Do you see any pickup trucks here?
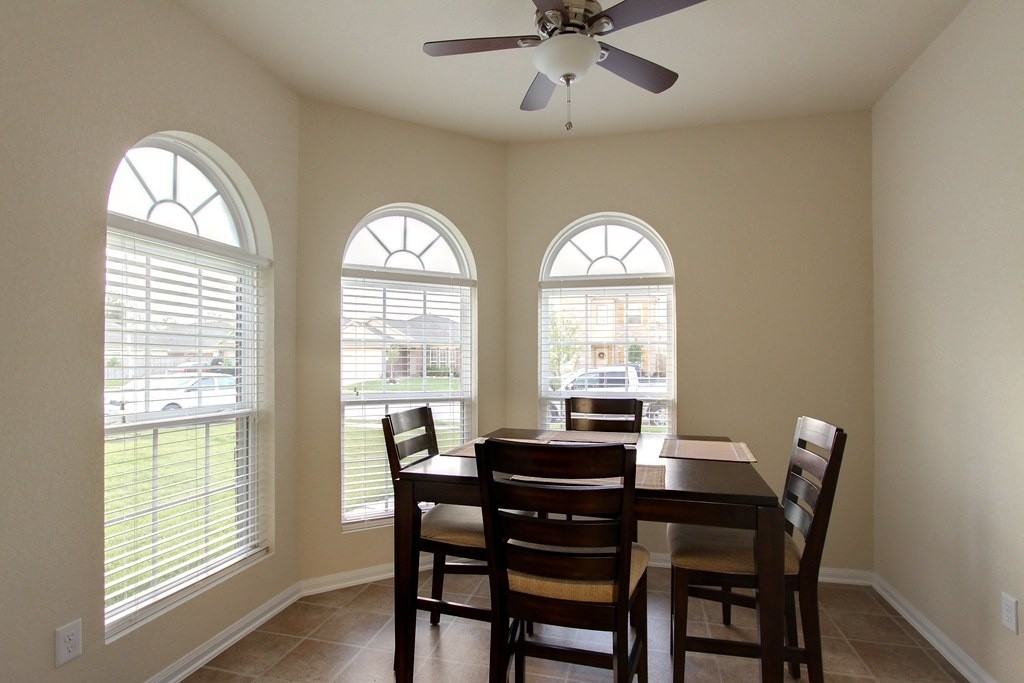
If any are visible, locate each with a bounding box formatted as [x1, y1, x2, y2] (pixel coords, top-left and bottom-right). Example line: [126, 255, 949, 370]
[548, 366, 673, 427]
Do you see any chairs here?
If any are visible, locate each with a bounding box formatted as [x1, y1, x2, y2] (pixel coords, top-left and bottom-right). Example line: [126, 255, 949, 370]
[473, 437, 650, 683]
[382, 407, 533, 670]
[666, 416, 847, 683]
[538, 395, 643, 542]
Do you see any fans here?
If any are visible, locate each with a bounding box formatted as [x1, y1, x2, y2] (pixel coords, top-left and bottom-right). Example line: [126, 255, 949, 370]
[421, 0, 708, 111]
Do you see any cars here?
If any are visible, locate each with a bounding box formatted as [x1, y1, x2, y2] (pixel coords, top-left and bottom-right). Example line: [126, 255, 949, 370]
[124, 358, 235, 414]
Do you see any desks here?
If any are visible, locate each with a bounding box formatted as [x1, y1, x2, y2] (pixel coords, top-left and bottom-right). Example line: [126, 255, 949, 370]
[396, 427, 786, 683]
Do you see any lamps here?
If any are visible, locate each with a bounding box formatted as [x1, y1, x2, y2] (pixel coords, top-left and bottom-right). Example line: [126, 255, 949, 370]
[533, 33, 601, 132]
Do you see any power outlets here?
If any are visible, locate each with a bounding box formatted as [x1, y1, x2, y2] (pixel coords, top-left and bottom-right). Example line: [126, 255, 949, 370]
[1001, 592, 1018, 633]
